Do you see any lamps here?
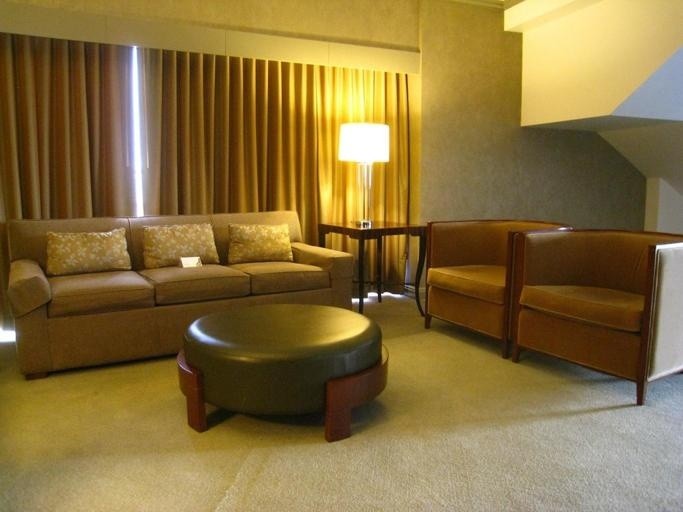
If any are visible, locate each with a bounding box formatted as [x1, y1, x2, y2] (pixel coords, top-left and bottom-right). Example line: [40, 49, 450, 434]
[339, 124, 391, 224]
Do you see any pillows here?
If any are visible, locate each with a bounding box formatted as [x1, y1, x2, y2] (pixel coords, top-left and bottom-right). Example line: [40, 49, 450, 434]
[45, 225, 131, 276]
[227, 222, 295, 263]
[142, 221, 220, 269]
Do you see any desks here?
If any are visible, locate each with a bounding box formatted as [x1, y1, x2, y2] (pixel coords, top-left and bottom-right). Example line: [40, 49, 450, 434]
[175, 302, 391, 444]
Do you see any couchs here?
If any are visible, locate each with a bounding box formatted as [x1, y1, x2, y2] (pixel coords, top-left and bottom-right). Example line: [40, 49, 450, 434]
[426, 218, 569, 358]
[510, 227, 682, 406]
[7, 210, 355, 381]
